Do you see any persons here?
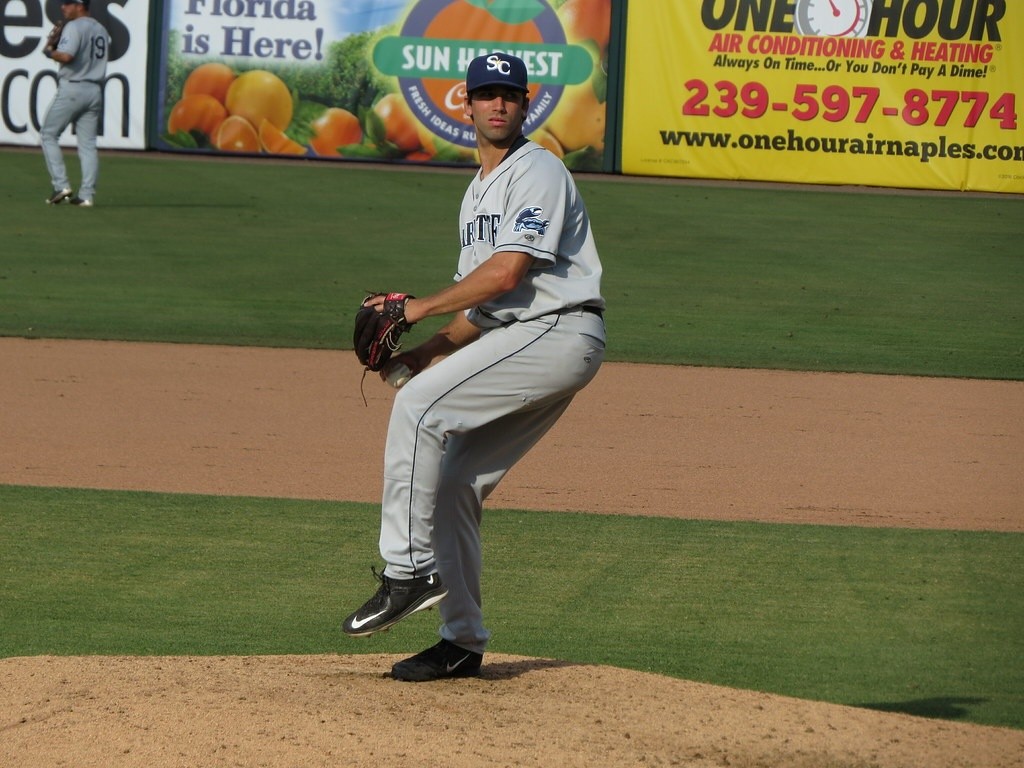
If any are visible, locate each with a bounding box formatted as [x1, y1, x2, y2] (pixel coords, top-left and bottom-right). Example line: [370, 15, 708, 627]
[341, 53, 607, 681]
[39, 0, 111, 208]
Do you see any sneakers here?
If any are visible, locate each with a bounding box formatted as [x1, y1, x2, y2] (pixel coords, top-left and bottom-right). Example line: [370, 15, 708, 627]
[71, 195, 94, 208]
[45, 187, 72, 204]
[342, 566, 449, 637]
[392, 638, 483, 682]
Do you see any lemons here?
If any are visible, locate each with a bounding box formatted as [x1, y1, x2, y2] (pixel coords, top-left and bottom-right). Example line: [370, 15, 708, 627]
[525, 72, 605, 157]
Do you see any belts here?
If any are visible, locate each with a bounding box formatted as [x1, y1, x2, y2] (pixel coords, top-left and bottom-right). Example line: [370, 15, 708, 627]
[69, 79, 99, 83]
[580, 304, 602, 317]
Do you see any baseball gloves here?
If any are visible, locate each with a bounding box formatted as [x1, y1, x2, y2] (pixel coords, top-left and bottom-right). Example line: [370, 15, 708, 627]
[353, 292, 417, 371]
[45, 22, 64, 49]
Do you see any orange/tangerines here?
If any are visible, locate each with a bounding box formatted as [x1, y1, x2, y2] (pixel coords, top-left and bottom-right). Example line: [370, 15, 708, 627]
[167, 63, 422, 156]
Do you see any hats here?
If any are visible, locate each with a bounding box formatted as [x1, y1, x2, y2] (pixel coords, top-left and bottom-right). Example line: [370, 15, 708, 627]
[466, 52, 530, 93]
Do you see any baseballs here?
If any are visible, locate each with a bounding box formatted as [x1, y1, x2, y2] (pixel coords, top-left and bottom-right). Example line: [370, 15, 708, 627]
[384, 363, 411, 389]
